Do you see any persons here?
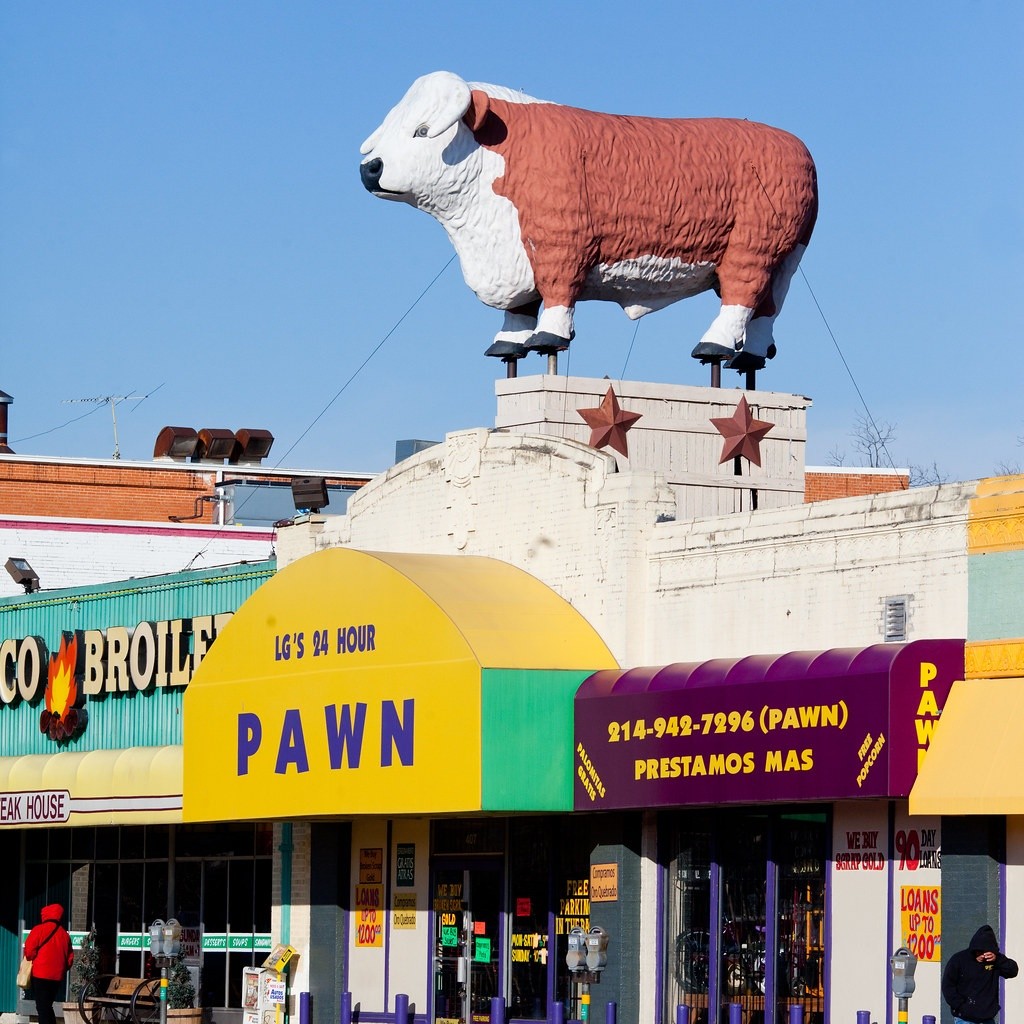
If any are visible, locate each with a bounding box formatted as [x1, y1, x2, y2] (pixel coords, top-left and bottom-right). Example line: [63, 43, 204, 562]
[941, 924, 1019, 1024]
[24, 904, 74, 1024]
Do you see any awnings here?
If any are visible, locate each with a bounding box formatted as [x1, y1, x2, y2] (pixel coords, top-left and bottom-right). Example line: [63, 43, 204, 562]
[908, 678, 1024, 816]
[0, 745, 183, 829]
[178, 545, 621, 824]
[572, 636, 965, 811]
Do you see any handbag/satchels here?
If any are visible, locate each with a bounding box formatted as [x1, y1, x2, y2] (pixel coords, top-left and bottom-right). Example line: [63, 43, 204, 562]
[16, 956, 32, 989]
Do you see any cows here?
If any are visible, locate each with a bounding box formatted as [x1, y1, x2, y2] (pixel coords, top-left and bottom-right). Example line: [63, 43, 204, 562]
[360, 69, 820, 370]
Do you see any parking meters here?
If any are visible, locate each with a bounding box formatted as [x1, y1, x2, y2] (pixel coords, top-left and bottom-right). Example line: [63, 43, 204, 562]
[569, 926, 610, 1023]
[148, 919, 185, 1023]
[889, 948, 917, 1024]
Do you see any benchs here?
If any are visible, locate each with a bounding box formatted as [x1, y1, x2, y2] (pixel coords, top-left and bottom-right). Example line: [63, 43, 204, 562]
[80, 974, 171, 1024]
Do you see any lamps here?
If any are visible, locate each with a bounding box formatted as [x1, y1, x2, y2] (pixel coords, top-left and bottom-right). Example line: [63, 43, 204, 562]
[235, 428, 275, 458]
[192, 428, 238, 459]
[4, 557, 40, 595]
[31, 578, 41, 593]
[291, 476, 330, 514]
[153, 425, 199, 458]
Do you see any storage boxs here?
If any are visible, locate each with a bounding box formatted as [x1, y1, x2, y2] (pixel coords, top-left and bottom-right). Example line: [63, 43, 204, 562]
[261, 944, 296, 973]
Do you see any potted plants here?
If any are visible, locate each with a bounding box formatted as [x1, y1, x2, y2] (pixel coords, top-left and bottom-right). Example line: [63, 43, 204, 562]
[166, 962, 202, 1024]
[65, 927, 103, 1024]
